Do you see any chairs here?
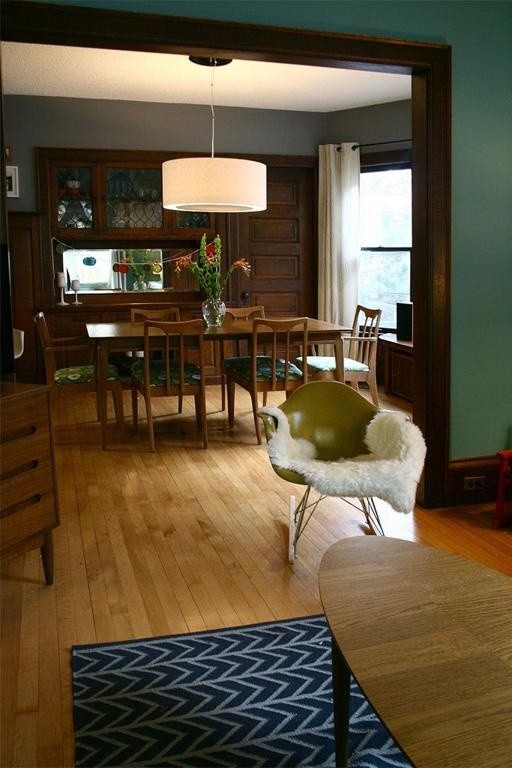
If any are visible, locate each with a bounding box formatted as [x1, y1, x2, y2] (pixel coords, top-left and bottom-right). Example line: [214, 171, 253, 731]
[12, 328, 25, 382]
[254, 379, 428, 565]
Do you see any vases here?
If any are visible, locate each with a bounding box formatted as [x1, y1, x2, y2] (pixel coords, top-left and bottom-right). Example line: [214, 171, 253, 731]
[132, 274, 146, 290]
[201, 288, 225, 327]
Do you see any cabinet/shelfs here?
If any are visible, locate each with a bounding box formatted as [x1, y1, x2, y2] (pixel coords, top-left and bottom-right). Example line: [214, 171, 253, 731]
[378, 332, 414, 403]
[227, 152, 319, 375]
[37, 302, 239, 389]
[34, 146, 216, 241]
[0, 382, 61, 584]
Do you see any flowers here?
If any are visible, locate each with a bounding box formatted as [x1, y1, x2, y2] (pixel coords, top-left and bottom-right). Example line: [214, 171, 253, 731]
[173, 232, 252, 323]
[118, 248, 158, 289]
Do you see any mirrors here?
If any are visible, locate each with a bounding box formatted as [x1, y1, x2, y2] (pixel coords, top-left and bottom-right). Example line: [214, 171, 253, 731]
[62, 247, 164, 294]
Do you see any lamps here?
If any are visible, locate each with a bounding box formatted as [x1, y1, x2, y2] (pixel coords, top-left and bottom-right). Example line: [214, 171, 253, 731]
[161, 54, 268, 213]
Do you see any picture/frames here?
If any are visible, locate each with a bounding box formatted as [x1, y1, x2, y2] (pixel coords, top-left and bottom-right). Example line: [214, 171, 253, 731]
[5, 165, 19, 198]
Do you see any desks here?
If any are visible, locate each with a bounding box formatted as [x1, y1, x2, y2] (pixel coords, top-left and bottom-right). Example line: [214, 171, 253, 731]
[317, 534, 512, 767]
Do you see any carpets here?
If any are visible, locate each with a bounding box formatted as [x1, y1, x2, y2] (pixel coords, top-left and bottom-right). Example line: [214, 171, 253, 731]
[70, 613, 417, 767]
